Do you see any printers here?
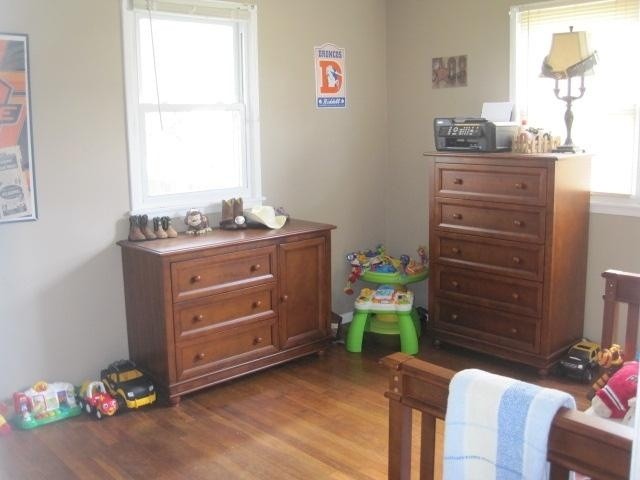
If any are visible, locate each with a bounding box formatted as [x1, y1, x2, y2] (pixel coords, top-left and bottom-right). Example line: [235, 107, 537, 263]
[434, 113, 520, 152]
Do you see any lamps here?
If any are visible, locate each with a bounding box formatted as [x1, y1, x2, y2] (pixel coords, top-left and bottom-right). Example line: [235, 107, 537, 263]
[537, 25, 598, 150]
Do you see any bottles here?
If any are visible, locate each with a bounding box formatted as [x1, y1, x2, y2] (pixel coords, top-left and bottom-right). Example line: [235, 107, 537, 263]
[519, 119, 530, 154]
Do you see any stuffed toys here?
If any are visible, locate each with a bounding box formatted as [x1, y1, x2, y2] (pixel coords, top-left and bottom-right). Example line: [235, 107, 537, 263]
[587, 343, 622, 400]
[184, 210, 212, 234]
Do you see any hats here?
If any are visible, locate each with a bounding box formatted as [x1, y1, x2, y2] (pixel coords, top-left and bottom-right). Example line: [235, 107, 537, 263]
[246, 206, 287, 229]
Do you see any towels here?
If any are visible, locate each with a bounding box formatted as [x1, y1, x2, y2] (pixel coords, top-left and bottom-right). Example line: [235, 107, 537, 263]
[441, 369, 577, 480]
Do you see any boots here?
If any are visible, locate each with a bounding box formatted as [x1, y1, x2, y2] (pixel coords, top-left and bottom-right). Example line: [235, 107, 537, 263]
[128, 216, 146, 240]
[234, 198, 247, 228]
[162, 217, 177, 238]
[220, 199, 238, 229]
[153, 217, 168, 239]
[138, 215, 157, 240]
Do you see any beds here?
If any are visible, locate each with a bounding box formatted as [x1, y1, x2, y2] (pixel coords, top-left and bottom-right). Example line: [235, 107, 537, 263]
[379, 267, 639, 480]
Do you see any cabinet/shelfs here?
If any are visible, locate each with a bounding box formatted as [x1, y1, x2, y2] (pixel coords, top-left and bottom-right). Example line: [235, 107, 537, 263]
[423, 151, 593, 379]
[116, 219, 338, 407]
